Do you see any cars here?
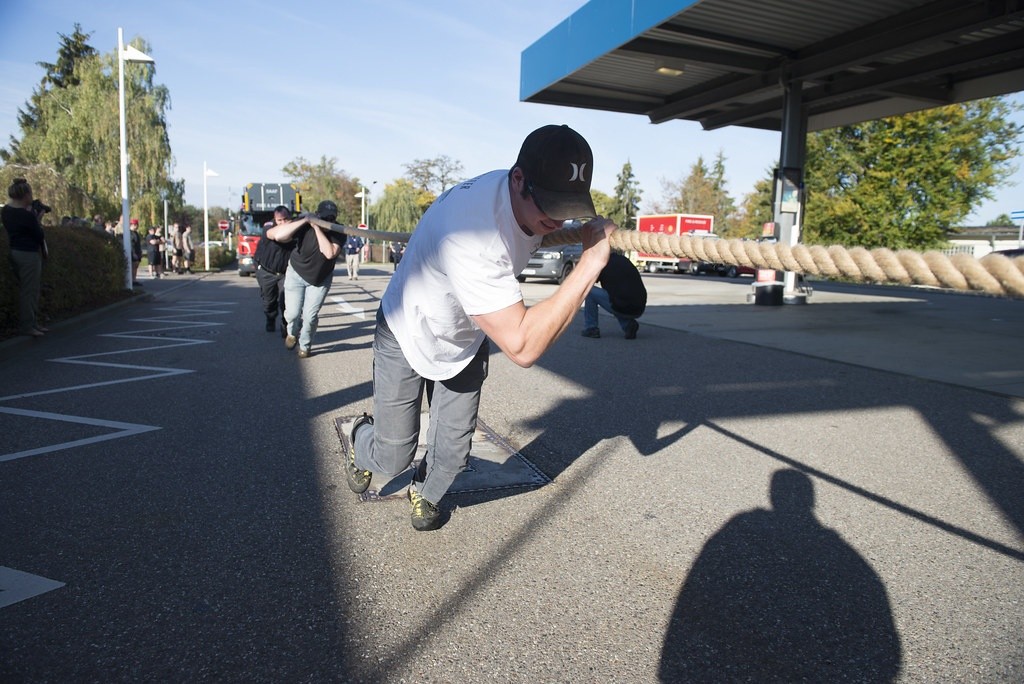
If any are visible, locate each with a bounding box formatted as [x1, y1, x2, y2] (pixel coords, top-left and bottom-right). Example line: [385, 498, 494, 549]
[198, 240, 226, 248]
[685, 257, 755, 278]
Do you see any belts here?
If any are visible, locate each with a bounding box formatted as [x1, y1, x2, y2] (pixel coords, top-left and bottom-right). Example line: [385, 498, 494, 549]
[256, 261, 282, 276]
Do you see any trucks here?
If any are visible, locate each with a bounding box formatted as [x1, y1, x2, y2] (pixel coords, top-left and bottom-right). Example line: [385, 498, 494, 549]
[236, 182, 304, 278]
[623, 213, 714, 273]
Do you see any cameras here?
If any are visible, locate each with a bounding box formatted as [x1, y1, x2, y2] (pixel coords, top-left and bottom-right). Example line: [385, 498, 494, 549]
[33, 199, 52, 213]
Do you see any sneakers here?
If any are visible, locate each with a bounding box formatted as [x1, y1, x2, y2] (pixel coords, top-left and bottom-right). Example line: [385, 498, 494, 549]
[346, 412, 375, 493]
[407, 481, 440, 530]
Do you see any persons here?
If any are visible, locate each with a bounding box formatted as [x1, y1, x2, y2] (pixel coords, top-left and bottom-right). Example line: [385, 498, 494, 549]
[253, 206, 311, 338]
[145, 227, 164, 279]
[342, 233, 363, 280]
[92, 214, 106, 234]
[274, 200, 348, 358]
[80, 218, 91, 228]
[113, 213, 123, 246]
[104, 219, 115, 237]
[389, 239, 407, 274]
[580, 246, 647, 339]
[171, 222, 183, 275]
[0, 178, 49, 336]
[182, 225, 195, 274]
[335, 123, 619, 526]
[71, 215, 81, 228]
[60, 216, 71, 228]
[155, 225, 169, 276]
[129, 217, 143, 286]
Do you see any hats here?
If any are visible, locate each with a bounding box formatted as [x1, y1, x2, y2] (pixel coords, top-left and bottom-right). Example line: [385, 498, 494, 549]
[318, 201, 339, 219]
[517, 124, 598, 220]
[130, 220, 138, 224]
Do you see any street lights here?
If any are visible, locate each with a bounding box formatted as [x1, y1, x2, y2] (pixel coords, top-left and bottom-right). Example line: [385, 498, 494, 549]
[354, 187, 366, 224]
[117, 26, 155, 293]
[203, 161, 220, 272]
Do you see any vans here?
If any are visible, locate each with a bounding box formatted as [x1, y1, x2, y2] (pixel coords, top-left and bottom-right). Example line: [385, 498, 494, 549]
[516, 223, 593, 286]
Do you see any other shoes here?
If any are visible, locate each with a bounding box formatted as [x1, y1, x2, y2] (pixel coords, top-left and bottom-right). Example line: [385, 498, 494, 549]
[281, 321, 288, 337]
[286, 334, 297, 349]
[581, 327, 600, 338]
[152, 270, 195, 279]
[623, 319, 639, 341]
[299, 350, 311, 359]
[266, 316, 278, 331]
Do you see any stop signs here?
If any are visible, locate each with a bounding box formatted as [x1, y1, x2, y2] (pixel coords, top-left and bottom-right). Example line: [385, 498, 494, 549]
[218, 219, 229, 230]
